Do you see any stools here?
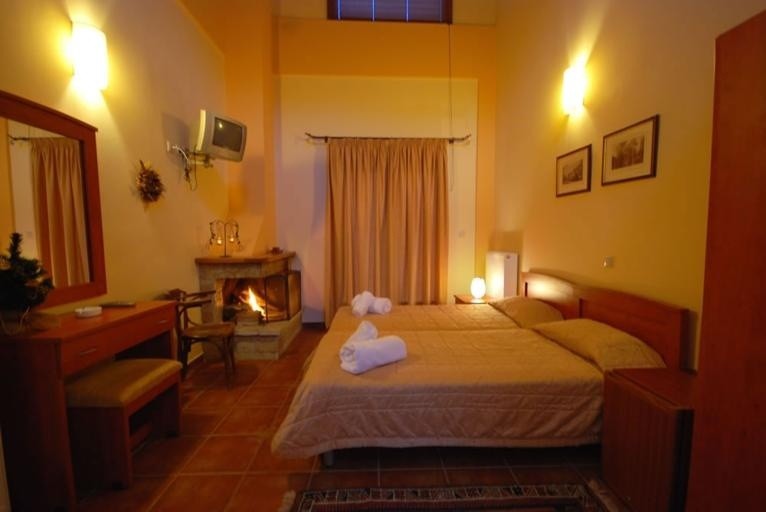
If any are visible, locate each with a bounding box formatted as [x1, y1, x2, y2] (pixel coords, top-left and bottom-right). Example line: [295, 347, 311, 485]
[66, 356, 184, 492]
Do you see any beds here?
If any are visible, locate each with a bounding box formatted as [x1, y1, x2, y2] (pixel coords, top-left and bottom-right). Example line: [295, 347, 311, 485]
[271, 286, 692, 469]
[327, 270, 576, 333]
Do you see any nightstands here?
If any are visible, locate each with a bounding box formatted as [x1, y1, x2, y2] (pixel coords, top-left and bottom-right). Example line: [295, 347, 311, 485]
[600, 365, 700, 511]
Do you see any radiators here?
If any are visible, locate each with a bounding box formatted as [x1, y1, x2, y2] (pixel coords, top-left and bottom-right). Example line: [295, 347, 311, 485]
[484, 251, 517, 301]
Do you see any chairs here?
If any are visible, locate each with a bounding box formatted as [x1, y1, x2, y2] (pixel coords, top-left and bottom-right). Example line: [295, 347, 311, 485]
[174, 289, 237, 382]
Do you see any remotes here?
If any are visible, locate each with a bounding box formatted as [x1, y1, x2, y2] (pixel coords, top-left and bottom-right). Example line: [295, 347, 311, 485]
[100, 301, 135, 307]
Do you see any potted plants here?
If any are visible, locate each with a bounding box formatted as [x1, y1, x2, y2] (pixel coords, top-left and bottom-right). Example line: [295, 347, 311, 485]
[0, 232, 56, 338]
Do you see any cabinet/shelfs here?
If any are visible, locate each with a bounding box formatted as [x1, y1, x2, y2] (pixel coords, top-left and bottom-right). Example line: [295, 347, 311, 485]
[683, 9, 765, 511]
[194, 250, 303, 361]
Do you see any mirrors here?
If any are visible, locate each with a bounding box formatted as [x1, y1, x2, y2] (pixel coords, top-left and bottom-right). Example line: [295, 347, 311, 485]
[0, 89, 108, 313]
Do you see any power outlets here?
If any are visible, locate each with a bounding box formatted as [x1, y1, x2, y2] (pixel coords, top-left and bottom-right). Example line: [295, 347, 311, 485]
[164, 139, 177, 154]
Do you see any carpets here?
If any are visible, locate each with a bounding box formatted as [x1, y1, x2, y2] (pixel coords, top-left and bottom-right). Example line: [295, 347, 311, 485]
[287, 479, 607, 511]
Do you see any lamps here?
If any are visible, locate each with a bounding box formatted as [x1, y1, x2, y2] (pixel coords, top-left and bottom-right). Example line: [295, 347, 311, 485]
[469, 275, 488, 305]
[208, 219, 242, 259]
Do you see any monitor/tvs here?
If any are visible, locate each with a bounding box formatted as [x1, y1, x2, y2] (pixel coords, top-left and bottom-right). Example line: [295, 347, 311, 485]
[187, 109, 247, 163]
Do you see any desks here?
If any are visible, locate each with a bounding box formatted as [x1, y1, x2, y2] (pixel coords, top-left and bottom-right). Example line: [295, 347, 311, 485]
[0, 299, 179, 512]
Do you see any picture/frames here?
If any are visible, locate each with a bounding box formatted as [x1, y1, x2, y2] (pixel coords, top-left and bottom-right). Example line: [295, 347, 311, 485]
[554, 142, 593, 199]
[600, 113, 661, 187]
